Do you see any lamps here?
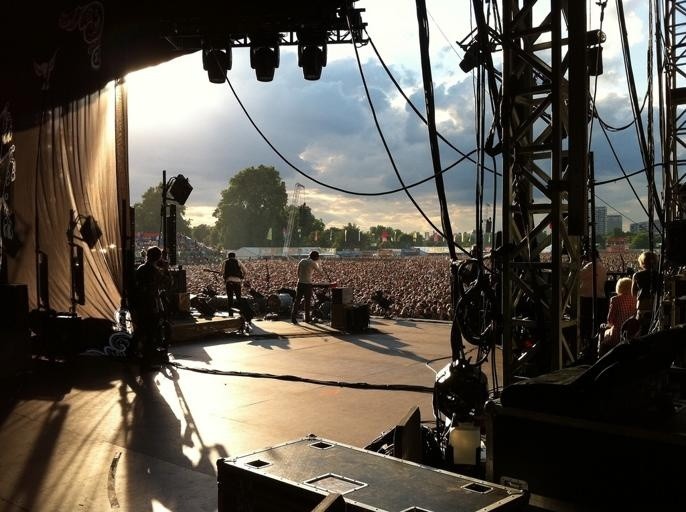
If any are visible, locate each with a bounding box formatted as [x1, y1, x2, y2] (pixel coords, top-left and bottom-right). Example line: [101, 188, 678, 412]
[457, 24, 504, 74]
[586, 26, 610, 83]
[194, 34, 336, 88]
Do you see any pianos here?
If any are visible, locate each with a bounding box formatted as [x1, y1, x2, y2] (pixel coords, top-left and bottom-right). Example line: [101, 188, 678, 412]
[312, 283, 337, 288]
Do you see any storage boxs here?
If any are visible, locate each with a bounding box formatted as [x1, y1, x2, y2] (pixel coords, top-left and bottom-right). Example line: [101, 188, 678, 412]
[211, 430, 531, 512]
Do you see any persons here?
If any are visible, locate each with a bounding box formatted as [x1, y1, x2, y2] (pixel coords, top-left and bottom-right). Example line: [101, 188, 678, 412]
[238, 251, 453, 320]
[168, 230, 226, 297]
[631, 251, 665, 336]
[577, 248, 607, 364]
[597, 278, 637, 360]
[129, 248, 173, 360]
[220, 252, 247, 317]
[134, 233, 158, 265]
[290, 250, 321, 323]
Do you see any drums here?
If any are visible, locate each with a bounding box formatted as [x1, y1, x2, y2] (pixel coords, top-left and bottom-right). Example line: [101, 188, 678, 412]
[267, 293, 292, 314]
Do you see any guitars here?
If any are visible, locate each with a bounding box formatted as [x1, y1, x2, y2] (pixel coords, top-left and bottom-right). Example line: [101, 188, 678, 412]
[204, 269, 242, 279]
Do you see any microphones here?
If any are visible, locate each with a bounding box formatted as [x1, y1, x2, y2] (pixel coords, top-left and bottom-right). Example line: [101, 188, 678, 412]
[261, 256, 269, 260]
